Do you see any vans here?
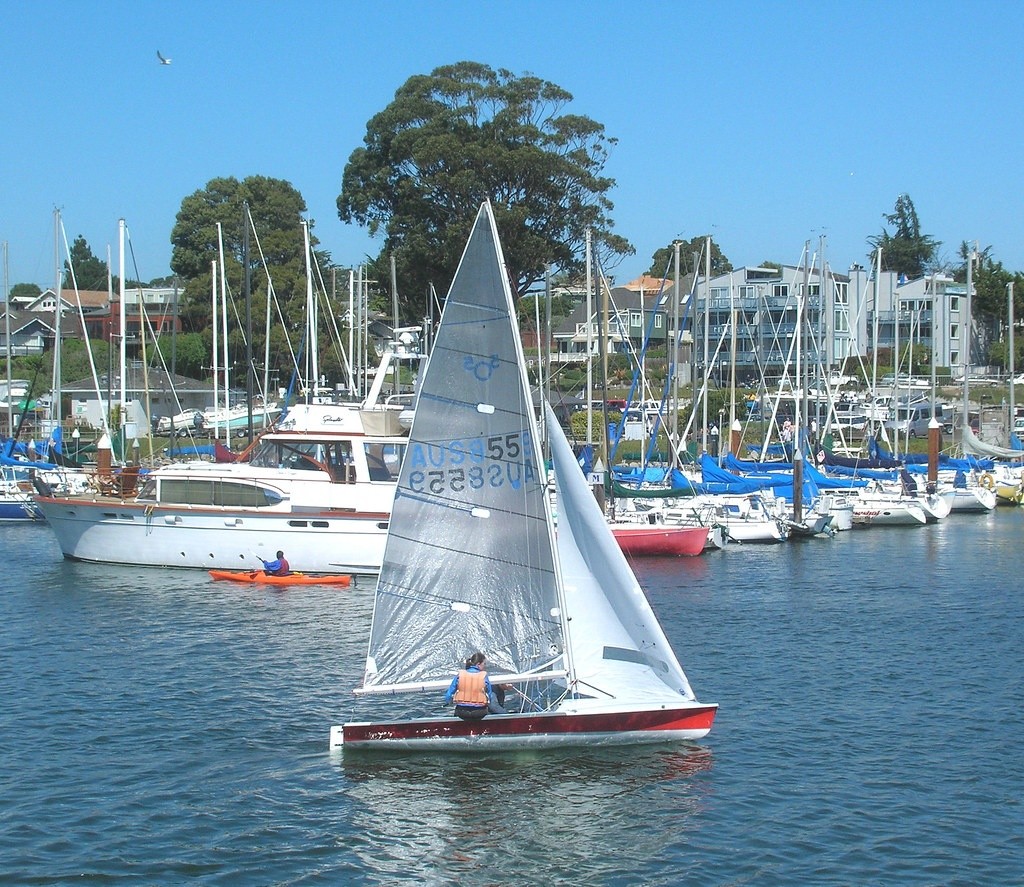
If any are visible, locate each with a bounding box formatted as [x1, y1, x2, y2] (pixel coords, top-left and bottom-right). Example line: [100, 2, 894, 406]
[883, 403, 944, 439]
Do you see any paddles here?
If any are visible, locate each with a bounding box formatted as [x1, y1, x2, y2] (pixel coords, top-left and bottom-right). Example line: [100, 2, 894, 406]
[248, 547, 264, 563]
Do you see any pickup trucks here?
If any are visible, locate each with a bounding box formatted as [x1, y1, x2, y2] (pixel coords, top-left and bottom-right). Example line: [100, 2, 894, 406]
[598, 399, 626, 409]
[592, 407, 642, 421]
[621, 401, 673, 416]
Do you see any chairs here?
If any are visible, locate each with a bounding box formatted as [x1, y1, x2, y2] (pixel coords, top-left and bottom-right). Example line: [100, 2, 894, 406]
[84, 466, 140, 496]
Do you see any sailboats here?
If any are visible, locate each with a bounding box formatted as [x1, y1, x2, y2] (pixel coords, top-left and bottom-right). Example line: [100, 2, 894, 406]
[534, 227, 1024, 555]
[1, 208, 443, 574]
[327, 196, 719, 749]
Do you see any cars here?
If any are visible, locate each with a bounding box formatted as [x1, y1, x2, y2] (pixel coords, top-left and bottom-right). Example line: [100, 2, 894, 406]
[944, 412, 979, 434]
[818, 370, 859, 386]
[1006, 372, 1024, 386]
[823, 415, 870, 437]
[880, 372, 929, 386]
[954, 373, 1003, 389]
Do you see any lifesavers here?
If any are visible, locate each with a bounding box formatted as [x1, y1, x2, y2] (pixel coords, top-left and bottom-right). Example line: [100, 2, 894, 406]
[980, 473, 993, 488]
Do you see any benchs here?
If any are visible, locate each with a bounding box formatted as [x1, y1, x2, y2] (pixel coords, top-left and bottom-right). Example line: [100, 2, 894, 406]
[16, 482, 38, 498]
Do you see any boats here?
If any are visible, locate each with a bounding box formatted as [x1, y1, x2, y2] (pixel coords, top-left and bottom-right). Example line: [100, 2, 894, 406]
[210, 570, 351, 586]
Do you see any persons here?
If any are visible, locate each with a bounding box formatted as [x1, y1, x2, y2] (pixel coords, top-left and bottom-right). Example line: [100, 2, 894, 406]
[784, 417, 791, 428]
[810, 418, 816, 444]
[263, 551, 289, 576]
[488, 683, 514, 713]
[446, 653, 491, 718]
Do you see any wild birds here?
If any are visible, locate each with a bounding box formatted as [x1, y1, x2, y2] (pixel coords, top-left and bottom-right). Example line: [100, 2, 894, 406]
[156, 50, 172, 65]
